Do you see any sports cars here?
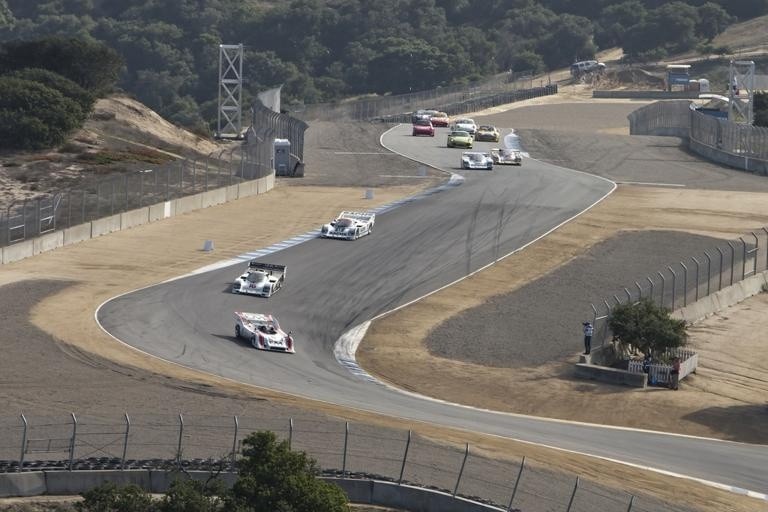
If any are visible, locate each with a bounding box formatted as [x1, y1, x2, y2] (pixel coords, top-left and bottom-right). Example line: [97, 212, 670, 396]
[461, 151, 494, 170]
[490, 148, 523, 166]
[231, 261, 288, 298]
[320, 210, 376, 241]
[232, 311, 296, 354]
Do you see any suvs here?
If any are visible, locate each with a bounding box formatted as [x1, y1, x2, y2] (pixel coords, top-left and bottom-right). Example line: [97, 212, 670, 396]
[570, 59, 606, 78]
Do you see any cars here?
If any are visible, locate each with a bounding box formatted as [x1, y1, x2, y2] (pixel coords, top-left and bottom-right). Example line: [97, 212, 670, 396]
[412, 120, 435, 137]
[447, 131, 474, 149]
[475, 125, 500, 142]
[453, 118, 476, 135]
[412, 109, 449, 127]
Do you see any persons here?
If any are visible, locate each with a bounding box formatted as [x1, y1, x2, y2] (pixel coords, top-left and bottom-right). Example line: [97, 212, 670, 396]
[583, 320, 593, 354]
